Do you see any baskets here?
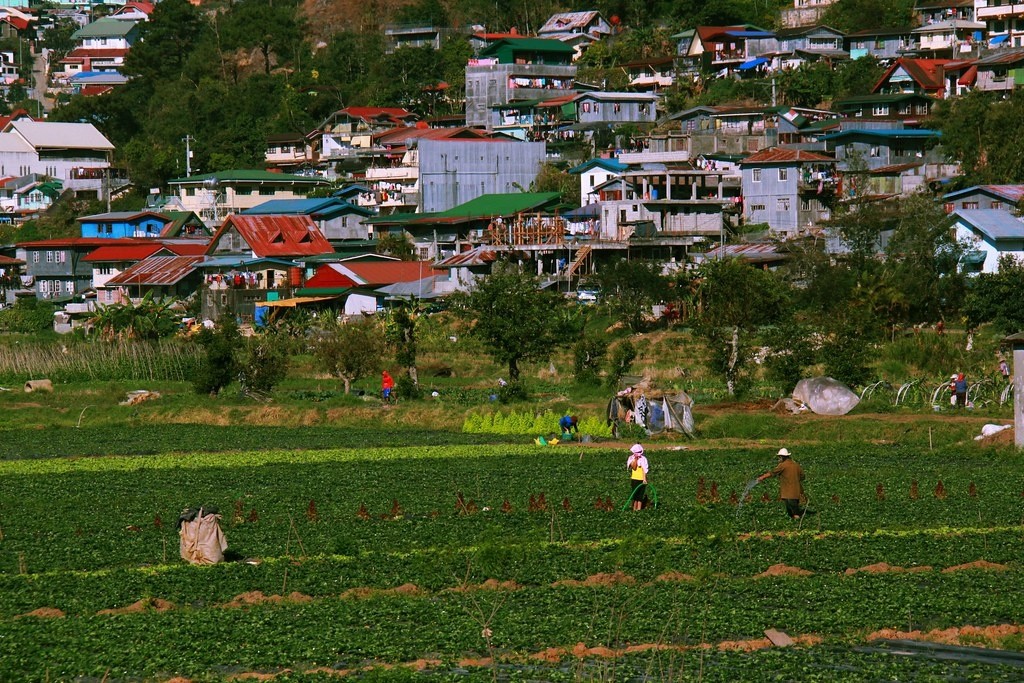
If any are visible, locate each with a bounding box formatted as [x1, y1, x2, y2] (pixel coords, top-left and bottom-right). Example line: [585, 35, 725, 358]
[562, 429, 572, 440]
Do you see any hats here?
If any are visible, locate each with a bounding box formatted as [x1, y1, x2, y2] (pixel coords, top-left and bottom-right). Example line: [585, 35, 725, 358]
[777, 448, 791, 456]
[999, 358, 1005, 362]
[631, 444, 643, 457]
[950, 374, 958, 379]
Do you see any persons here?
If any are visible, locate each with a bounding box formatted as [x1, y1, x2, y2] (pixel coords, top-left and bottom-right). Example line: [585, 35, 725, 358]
[560, 416, 578, 438]
[949, 374, 958, 409]
[966, 329, 973, 352]
[757, 448, 805, 518]
[799, 404, 808, 410]
[955, 372, 966, 407]
[627, 444, 649, 511]
[937, 320, 943, 334]
[1000, 356, 1010, 380]
[382, 371, 395, 404]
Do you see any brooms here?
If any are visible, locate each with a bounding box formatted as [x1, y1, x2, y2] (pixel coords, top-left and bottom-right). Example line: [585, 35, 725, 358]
[664, 395, 695, 441]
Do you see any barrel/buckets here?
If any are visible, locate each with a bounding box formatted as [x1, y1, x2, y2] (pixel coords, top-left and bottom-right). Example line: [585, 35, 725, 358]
[562, 434, 572, 440]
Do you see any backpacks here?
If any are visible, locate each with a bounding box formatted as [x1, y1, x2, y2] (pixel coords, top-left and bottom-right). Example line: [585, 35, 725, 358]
[1006, 367, 1010, 376]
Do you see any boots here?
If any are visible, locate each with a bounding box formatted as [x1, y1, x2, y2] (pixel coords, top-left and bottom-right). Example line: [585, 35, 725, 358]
[950, 404, 956, 411]
